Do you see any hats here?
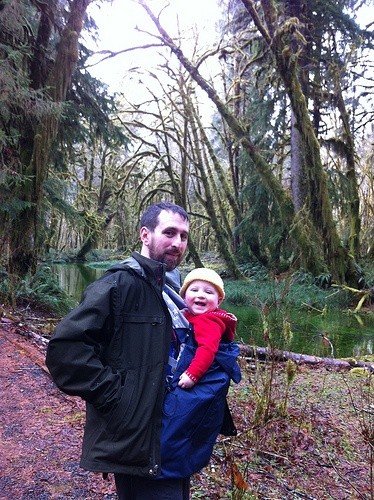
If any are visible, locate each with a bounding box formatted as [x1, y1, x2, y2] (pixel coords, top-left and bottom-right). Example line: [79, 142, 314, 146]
[179, 268, 225, 305]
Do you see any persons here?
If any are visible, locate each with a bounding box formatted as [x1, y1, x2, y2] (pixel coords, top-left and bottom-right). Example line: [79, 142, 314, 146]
[178, 267, 237, 389]
[44, 203, 191, 499]
